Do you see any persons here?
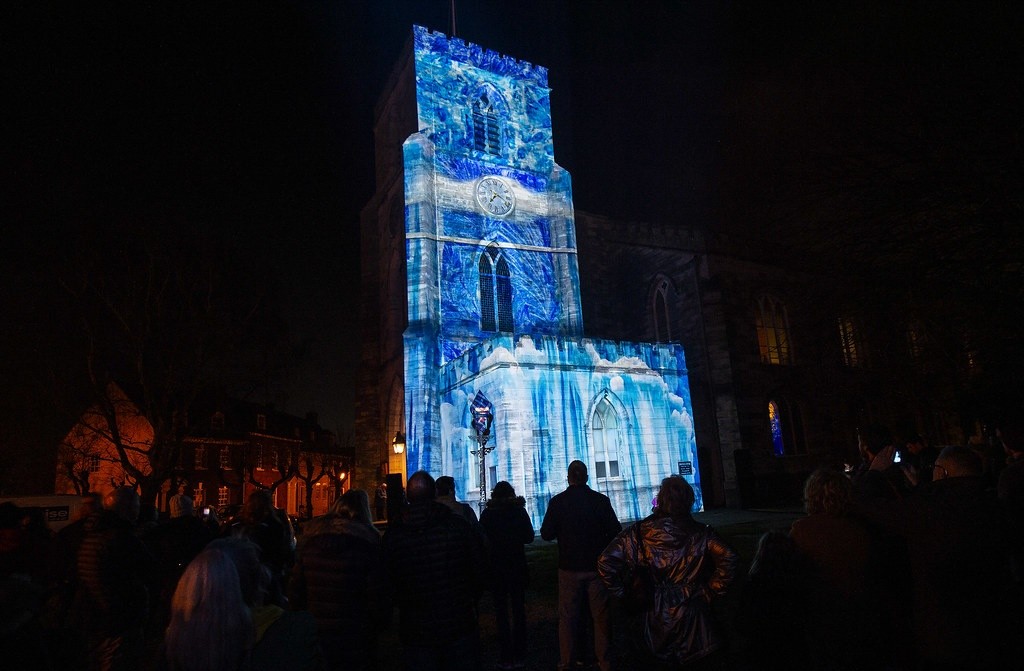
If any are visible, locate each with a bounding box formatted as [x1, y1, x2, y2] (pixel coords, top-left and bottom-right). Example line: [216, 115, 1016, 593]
[0, 469, 534, 671]
[597, 475, 738, 671]
[540, 461, 623, 671]
[737, 415, 1024, 671]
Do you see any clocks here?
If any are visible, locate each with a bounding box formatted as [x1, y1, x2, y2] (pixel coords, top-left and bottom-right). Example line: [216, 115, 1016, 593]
[475, 177, 514, 216]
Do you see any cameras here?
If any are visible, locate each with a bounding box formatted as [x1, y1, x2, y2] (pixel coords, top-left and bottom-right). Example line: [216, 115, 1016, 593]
[842, 462, 855, 473]
[892, 449, 904, 465]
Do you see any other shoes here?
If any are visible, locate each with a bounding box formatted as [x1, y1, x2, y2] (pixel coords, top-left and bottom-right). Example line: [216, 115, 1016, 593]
[594, 658, 609, 671]
[557, 662, 576, 671]
[513, 655, 525, 667]
[496, 656, 514, 669]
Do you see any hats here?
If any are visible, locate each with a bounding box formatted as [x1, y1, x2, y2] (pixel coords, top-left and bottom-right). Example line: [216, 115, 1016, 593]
[0, 502, 26, 530]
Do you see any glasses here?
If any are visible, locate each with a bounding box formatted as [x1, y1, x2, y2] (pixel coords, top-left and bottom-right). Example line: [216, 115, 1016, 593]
[929, 462, 948, 479]
[855, 423, 876, 436]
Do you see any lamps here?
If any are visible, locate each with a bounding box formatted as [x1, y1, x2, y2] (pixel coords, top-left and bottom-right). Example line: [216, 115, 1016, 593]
[392, 432, 405, 455]
